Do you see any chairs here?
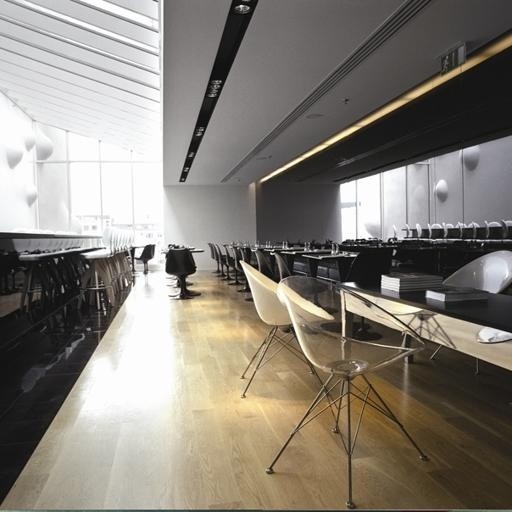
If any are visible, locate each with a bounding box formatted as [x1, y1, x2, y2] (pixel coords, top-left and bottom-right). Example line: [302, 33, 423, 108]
[11, 238, 100, 318]
